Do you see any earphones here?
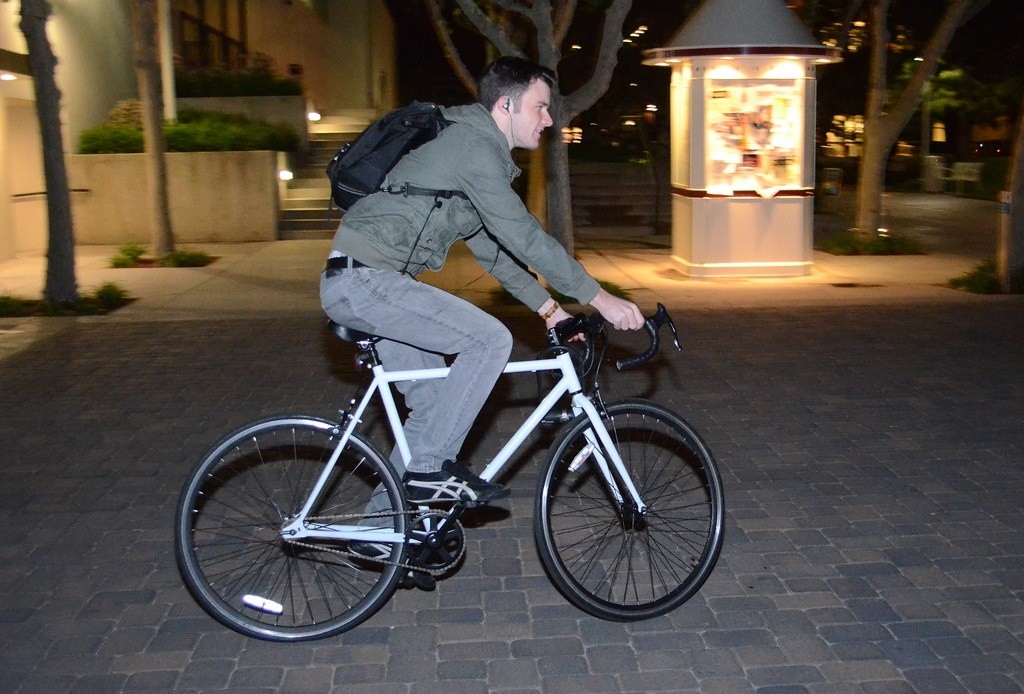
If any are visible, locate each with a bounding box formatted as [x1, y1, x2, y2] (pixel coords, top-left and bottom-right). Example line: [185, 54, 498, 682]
[503, 98, 510, 110]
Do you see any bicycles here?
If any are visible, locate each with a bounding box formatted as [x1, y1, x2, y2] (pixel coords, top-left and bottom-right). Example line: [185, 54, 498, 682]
[173, 302, 727, 641]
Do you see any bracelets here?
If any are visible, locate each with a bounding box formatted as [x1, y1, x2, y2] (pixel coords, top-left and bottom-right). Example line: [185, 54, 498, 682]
[539, 300, 559, 320]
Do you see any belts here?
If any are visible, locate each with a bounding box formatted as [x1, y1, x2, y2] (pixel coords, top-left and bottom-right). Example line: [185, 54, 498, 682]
[326, 257, 365, 268]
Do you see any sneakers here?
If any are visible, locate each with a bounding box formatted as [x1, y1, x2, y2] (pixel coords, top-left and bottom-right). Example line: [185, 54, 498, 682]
[401, 459, 511, 503]
[349, 539, 435, 589]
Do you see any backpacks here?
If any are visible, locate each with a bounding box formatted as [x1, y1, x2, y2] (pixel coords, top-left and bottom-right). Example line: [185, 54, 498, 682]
[325, 100, 469, 226]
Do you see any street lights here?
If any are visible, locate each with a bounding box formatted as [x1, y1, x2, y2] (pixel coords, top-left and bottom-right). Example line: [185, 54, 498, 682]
[914, 57, 932, 189]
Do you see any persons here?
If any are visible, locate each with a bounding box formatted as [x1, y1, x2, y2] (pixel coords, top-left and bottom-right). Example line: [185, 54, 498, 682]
[320, 56, 645, 560]
